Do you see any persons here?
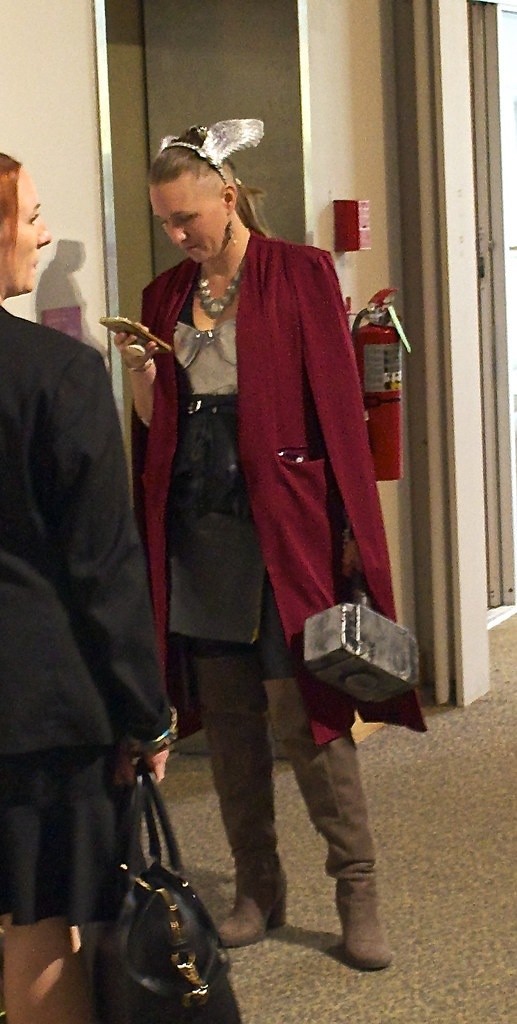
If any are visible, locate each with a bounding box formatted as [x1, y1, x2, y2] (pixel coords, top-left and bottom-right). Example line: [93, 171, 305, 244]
[116, 127, 428, 969]
[0, 152, 172, 1024]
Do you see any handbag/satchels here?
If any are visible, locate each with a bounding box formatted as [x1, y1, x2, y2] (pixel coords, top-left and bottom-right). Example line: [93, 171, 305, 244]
[92, 769, 243, 1024]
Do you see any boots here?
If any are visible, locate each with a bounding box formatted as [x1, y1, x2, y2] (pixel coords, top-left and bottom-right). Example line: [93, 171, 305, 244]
[263, 677, 392, 969]
[196, 661, 287, 946]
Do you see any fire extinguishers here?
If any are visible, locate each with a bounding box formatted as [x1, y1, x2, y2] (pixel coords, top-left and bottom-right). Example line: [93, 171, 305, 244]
[351, 287, 412, 482]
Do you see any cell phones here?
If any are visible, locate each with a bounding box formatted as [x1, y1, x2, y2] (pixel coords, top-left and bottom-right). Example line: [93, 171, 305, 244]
[100, 316, 171, 354]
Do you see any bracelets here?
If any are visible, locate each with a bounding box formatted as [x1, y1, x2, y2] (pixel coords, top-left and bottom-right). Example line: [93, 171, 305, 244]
[128, 361, 151, 373]
[134, 706, 178, 750]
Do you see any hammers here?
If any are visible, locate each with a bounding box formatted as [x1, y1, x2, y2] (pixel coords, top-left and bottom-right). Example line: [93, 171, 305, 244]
[301, 572, 421, 705]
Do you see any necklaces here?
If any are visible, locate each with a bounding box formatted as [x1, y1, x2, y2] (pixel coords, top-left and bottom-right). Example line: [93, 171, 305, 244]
[195, 263, 241, 319]
